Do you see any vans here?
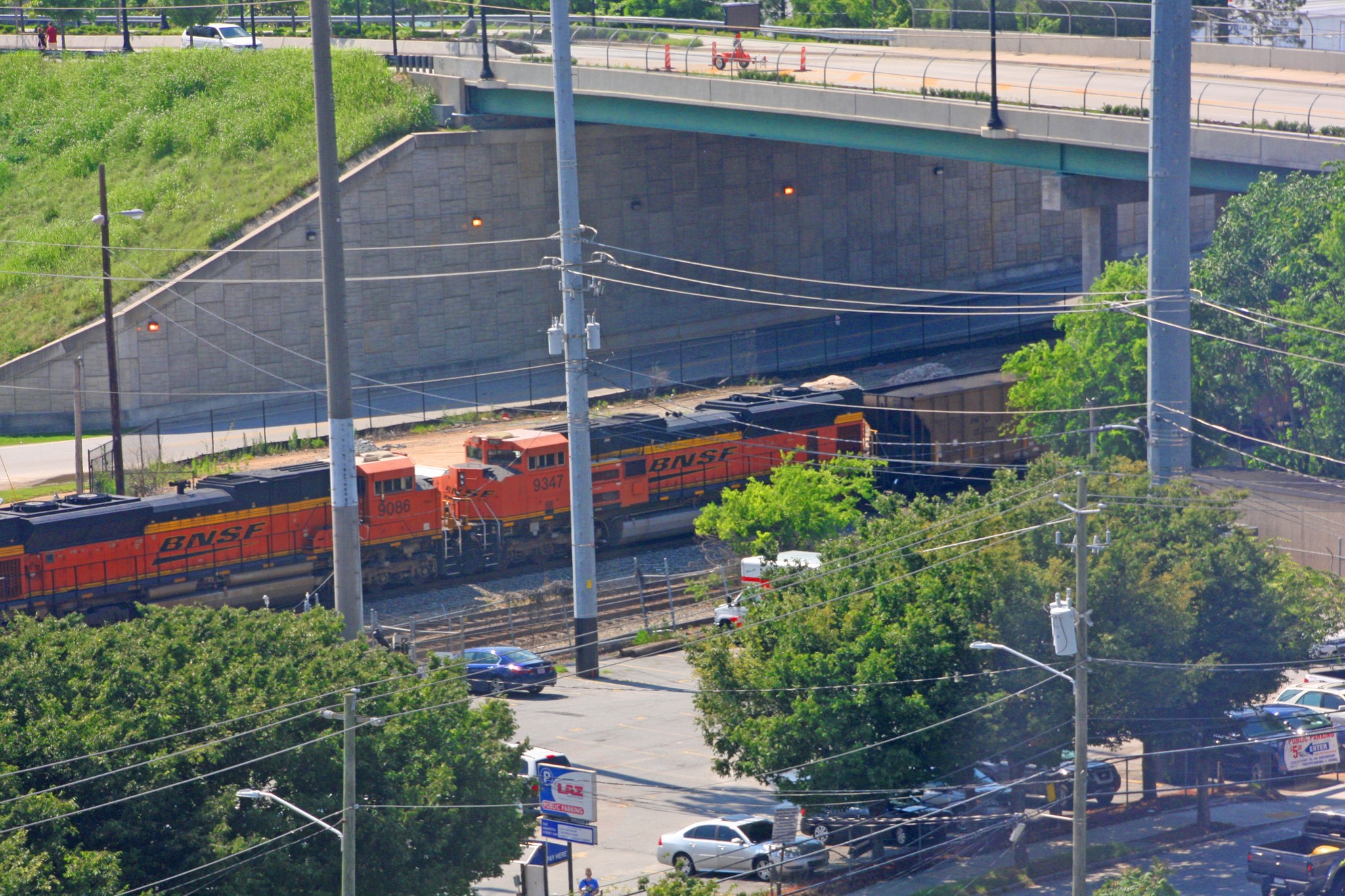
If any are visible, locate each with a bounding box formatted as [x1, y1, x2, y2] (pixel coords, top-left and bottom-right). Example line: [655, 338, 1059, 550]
[712, 549, 820, 637]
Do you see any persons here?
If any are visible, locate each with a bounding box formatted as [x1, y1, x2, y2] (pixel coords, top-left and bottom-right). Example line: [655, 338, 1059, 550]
[44, 22, 58, 50]
[578, 867, 599, 896]
[37, 27, 47, 50]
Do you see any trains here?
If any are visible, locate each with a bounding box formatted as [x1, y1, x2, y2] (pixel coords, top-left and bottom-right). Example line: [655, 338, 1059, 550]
[0, 368, 1039, 628]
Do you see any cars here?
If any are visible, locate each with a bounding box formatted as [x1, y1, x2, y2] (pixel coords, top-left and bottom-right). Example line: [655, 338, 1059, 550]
[417, 646, 557, 697]
[656, 813, 829, 883]
[1204, 702, 1337, 787]
[1303, 627, 1345, 658]
[1273, 679, 1345, 728]
[800, 801, 953, 846]
[897, 747, 1122, 834]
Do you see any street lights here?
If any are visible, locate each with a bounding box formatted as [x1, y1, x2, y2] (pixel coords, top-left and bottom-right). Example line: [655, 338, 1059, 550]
[968, 640, 1087, 896]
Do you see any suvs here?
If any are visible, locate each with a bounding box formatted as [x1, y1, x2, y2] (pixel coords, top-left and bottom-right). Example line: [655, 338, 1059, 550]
[500, 741, 574, 800]
[180, 22, 263, 53]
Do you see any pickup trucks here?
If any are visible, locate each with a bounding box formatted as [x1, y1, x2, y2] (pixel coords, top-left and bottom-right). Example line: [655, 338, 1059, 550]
[1246, 805, 1345, 896]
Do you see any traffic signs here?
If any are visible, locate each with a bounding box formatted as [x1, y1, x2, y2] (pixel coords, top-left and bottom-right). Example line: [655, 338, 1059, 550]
[1284, 730, 1340, 771]
